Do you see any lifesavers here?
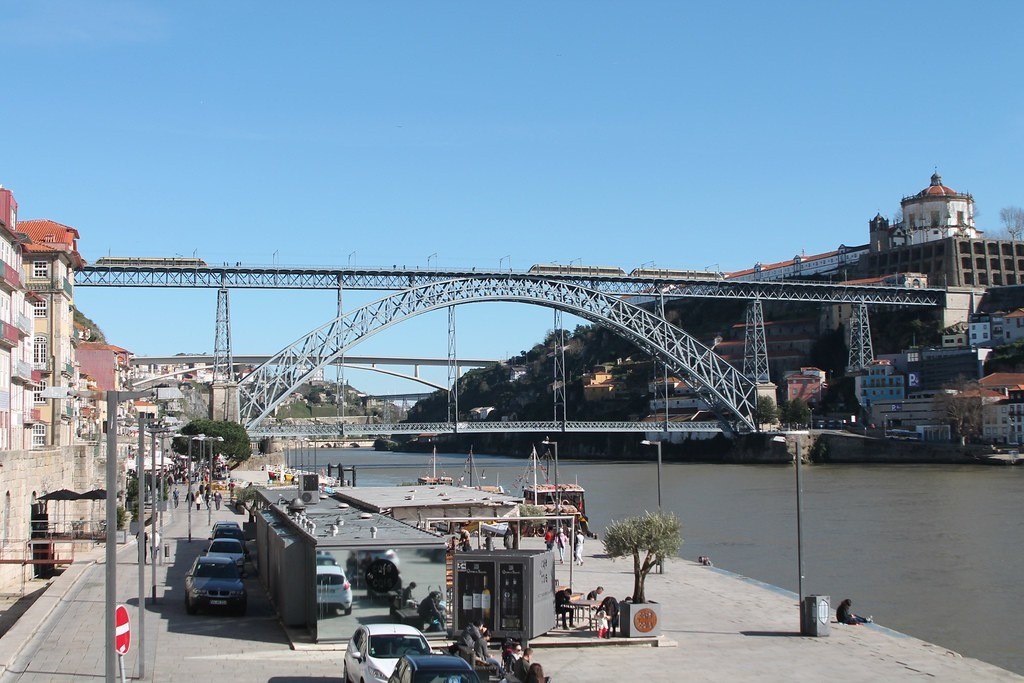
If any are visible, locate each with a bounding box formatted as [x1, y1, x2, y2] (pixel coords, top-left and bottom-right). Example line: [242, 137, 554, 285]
[562, 500, 569, 505]
[537, 527, 545, 536]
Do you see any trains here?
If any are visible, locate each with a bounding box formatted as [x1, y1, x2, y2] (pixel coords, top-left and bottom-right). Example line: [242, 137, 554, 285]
[96, 258, 208, 267]
[528, 264, 724, 281]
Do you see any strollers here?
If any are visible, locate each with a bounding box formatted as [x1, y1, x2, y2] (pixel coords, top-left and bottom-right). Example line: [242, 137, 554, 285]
[428, 585, 447, 617]
[501, 631, 522, 670]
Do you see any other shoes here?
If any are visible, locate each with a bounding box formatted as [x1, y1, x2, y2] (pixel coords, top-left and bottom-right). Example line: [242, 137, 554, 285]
[866, 614, 873, 622]
[563, 625, 569, 630]
[569, 624, 575, 627]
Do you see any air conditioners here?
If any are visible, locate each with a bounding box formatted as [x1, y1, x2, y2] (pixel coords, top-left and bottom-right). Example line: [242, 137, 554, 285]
[298, 491, 320, 504]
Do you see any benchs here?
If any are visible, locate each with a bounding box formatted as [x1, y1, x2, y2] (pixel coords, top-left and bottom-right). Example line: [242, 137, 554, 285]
[387, 589, 415, 622]
[453, 641, 499, 679]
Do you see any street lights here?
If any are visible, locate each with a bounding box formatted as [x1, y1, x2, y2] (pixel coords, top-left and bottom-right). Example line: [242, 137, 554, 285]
[641, 440, 664, 574]
[184, 433, 224, 542]
[39, 387, 186, 683]
[771, 436, 806, 635]
[294, 438, 310, 474]
[144, 426, 178, 606]
[156, 432, 183, 566]
[118, 416, 183, 680]
[542, 440, 558, 533]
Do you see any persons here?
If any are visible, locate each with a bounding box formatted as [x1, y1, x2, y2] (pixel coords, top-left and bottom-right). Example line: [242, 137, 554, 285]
[546, 526, 584, 566]
[503, 527, 513, 548]
[417, 590, 447, 630]
[343, 549, 376, 588]
[587, 586, 606, 611]
[149, 529, 160, 562]
[555, 588, 575, 630]
[460, 620, 551, 683]
[403, 582, 418, 606]
[596, 596, 619, 639]
[447, 527, 472, 551]
[136, 528, 148, 565]
[595, 611, 611, 639]
[127, 454, 235, 510]
[836, 599, 873, 624]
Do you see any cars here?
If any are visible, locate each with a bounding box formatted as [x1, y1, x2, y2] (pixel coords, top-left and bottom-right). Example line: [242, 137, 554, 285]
[388, 654, 481, 683]
[185, 554, 246, 615]
[342, 623, 434, 683]
[316, 550, 400, 615]
[202, 520, 248, 575]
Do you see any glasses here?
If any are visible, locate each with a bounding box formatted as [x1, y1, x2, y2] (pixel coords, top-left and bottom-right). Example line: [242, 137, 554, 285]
[516, 649, 522, 652]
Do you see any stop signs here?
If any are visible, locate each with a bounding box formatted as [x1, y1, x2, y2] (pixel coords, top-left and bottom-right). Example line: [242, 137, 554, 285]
[115, 605, 131, 656]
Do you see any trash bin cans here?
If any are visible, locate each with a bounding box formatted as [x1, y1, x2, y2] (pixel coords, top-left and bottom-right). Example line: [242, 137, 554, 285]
[804, 596, 831, 637]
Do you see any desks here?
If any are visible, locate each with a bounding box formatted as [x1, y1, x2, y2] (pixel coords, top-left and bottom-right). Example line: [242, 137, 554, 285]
[573, 601, 602, 632]
[394, 607, 424, 631]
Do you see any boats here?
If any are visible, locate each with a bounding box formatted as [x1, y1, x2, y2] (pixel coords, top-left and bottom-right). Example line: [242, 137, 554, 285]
[418, 443, 586, 534]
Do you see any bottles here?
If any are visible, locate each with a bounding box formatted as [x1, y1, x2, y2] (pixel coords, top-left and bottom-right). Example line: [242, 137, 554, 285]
[463, 575, 519, 628]
